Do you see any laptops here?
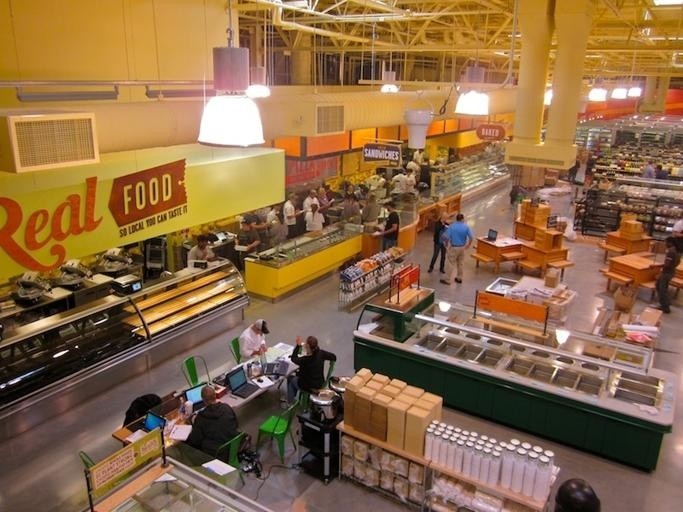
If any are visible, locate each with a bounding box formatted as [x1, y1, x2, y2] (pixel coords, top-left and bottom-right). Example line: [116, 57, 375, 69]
[485, 229, 497, 241]
[141, 409, 166, 433]
[184, 382, 207, 414]
[258, 348, 288, 376]
[227, 366, 259, 399]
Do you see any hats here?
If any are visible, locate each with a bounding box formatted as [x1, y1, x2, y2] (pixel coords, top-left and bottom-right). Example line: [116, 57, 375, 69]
[254, 318, 271, 334]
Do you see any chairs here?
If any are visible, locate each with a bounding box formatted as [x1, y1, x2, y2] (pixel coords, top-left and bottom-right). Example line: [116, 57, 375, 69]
[256, 400, 299, 464]
[229, 337, 242, 363]
[78, 450, 95, 469]
[216, 431, 246, 486]
[181, 355, 210, 387]
[302, 361, 335, 413]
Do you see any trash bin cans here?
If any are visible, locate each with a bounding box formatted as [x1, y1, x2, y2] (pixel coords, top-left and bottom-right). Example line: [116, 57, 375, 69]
[326, 207, 345, 224]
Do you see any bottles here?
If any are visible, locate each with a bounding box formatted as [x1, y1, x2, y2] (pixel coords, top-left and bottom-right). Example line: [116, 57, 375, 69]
[423, 420, 553, 505]
[589, 154, 643, 182]
[246, 363, 252, 379]
[177, 396, 189, 422]
[259, 348, 266, 368]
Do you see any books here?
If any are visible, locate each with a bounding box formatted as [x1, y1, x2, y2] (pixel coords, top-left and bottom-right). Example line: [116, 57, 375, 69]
[169, 424, 193, 441]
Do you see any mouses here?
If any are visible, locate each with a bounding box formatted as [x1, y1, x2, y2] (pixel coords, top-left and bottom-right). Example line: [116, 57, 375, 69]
[257, 378, 264, 382]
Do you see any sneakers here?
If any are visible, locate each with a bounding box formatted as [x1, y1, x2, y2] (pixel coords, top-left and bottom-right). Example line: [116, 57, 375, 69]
[439, 268, 446, 274]
[455, 277, 462, 283]
[440, 279, 451, 285]
[427, 268, 434, 273]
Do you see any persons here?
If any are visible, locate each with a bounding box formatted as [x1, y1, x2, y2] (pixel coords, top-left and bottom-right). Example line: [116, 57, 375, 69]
[187, 234, 216, 263]
[568, 153, 587, 186]
[238, 318, 272, 364]
[587, 154, 596, 173]
[235, 145, 453, 274]
[672, 218, 682, 253]
[279, 334, 337, 410]
[438, 211, 473, 285]
[120, 391, 161, 450]
[639, 160, 656, 179]
[655, 164, 671, 179]
[655, 235, 681, 314]
[187, 386, 241, 458]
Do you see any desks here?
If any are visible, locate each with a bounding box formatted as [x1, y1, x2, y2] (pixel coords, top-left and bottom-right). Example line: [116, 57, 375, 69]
[112, 342, 307, 450]
[470, 220, 683, 303]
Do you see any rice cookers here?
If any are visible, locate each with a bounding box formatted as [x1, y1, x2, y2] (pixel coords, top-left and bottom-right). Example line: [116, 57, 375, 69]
[303, 385, 341, 421]
[327, 375, 351, 402]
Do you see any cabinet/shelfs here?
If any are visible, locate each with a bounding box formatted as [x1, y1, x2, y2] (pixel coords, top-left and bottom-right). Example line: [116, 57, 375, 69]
[0, 257, 250, 443]
[573, 111, 683, 242]
[335, 412, 561, 512]
[81, 453, 273, 512]
[431, 156, 511, 208]
[417, 192, 462, 232]
[338, 252, 408, 312]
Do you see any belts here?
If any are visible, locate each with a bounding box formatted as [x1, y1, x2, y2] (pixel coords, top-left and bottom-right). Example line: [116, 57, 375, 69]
[452, 244, 464, 247]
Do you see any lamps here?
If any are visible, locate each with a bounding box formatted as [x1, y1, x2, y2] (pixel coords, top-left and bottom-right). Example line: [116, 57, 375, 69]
[245, 65, 271, 99]
[588, 88, 641, 101]
[404, 96, 434, 149]
[380, 20, 398, 93]
[454, 1, 489, 115]
[197, 1, 268, 147]
